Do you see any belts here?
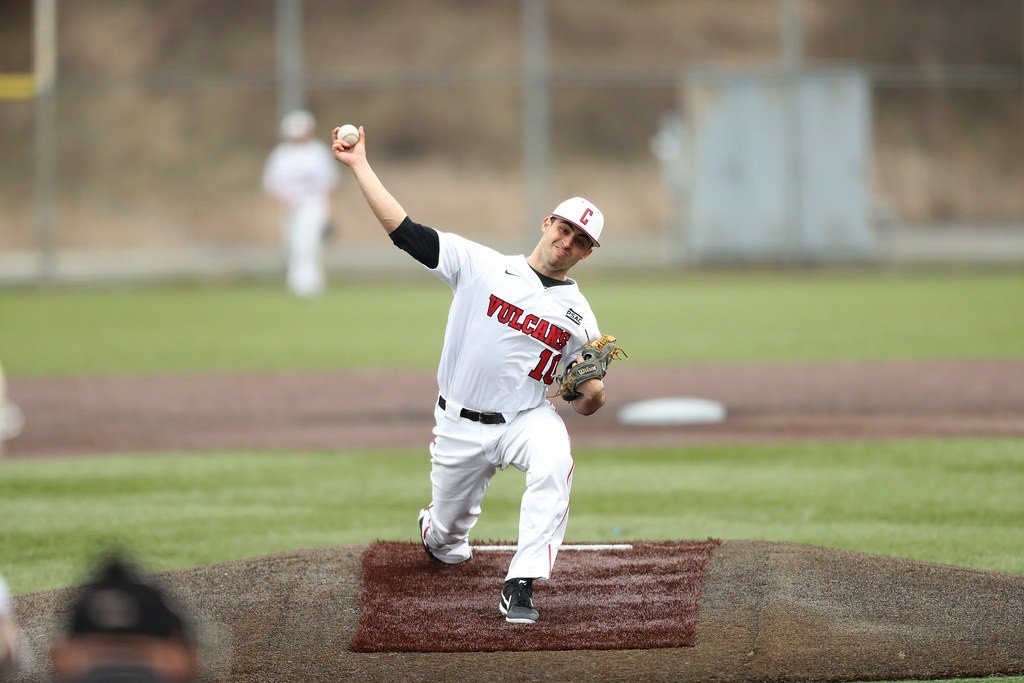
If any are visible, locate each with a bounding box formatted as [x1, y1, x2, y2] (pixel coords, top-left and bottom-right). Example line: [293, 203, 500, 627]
[438, 396, 506, 424]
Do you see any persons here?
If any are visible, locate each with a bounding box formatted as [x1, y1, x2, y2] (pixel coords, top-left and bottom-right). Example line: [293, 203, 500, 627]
[319, 122, 607, 624]
[40, 542, 204, 682]
[262, 109, 338, 297]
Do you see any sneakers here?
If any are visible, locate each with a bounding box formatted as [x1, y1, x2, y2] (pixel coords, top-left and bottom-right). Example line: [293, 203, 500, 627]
[418, 510, 442, 562]
[498, 577, 539, 623]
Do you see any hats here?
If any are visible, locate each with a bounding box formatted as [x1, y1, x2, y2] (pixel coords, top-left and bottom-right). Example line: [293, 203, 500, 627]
[551, 197, 605, 248]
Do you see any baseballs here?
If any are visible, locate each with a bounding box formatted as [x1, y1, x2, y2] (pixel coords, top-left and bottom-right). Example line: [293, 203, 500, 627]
[337, 124, 359, 147]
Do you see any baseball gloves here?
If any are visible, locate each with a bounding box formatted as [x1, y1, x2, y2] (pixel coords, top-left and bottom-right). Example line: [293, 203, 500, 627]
[555, 334, 618, 402]
